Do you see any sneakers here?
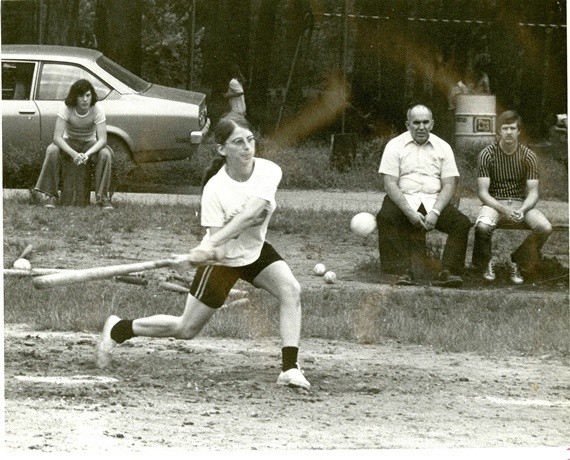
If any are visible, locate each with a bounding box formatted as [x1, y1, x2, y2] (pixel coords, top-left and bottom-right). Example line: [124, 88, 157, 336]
[97, 196, 114, 210]
[503, 254, 524, 284]
[42, 197, 55, 209]
[276, 361, 310, 390]
[483, 256, 496, 280]
[97, 315, 122, 368]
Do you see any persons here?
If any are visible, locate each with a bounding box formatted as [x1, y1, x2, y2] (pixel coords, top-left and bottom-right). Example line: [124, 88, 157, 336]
[218, 74, 250, 132]
[33, 78, 117, 211]
[469, 111, 554, 283]
[375, 103, 469, 287]
[92, 119, 313, 393]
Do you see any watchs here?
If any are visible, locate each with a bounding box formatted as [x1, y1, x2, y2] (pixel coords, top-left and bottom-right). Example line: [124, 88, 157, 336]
[428, 206, 443, 217]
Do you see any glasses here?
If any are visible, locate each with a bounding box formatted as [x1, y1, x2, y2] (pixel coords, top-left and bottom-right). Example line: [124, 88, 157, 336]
[225, 136, 255, 145]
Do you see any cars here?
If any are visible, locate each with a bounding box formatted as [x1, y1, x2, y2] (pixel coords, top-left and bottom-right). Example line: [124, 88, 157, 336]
[1, 45, 211, 191]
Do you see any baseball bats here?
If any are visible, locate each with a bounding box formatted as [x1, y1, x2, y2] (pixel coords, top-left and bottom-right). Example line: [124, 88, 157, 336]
[32, 249, 224, 290]
[19, 244, 32, 258]
[218, 299, 250, 313]
[4, 269, 69, 275]
[172, 273, 248, 297]
[159, 282, 188, 294]
[3, 268, 147, 285]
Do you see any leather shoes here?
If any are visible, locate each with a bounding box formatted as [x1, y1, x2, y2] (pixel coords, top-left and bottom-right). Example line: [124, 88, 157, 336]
[396, 267, 415, 284]
[431, 272, 463, 288]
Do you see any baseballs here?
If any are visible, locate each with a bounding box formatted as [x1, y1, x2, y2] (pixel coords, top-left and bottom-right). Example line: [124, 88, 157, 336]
[13, 258, 31, 269]
[323, 271, 336, 284]
[350, 212, 377, 238]
[314, 264, 326, 276]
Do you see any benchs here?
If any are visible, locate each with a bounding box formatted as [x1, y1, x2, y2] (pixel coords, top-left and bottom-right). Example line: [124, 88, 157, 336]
[409, 214, 569, 278]
